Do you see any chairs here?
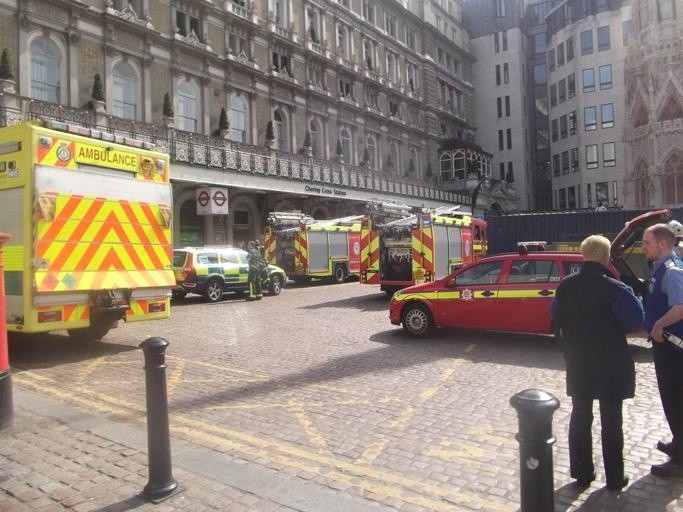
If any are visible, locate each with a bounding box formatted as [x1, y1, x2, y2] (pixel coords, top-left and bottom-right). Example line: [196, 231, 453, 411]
[506, 267, 530, 281]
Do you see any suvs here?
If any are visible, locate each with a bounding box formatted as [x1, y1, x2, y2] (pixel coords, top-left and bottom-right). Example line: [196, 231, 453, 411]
[171, 245, 287, 302]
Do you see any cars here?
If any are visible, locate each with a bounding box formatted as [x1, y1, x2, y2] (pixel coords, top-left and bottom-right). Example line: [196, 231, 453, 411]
[389, 209, 670, 337]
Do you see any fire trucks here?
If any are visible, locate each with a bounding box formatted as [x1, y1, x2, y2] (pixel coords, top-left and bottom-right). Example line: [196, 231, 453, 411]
[263, 209, 365, 285]
[376, 205, 490, 297]
[0, 112, 172, 349]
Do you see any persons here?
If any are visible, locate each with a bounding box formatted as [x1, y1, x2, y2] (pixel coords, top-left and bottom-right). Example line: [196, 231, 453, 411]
[641, 224, 683, 478]
[551, 235, 645, 489]
[595, 203, 609, 212]
[647, 220, 683, 270]
[246, 240, 262, 300]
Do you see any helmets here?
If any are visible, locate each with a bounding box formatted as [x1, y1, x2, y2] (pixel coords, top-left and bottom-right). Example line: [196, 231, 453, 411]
[668, 219, 683, 239]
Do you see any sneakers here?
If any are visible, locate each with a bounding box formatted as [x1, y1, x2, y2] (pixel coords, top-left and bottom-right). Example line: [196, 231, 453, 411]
[246, 297, 254, 301]
[255, 296, 261, 300]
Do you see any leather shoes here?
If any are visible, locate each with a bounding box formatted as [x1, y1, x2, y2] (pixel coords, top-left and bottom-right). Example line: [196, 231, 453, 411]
[657, 440, 672, 458]
[607, 473, 629, 490]
[651, 460, 683, 478]
[577, 471, 596, 489]
